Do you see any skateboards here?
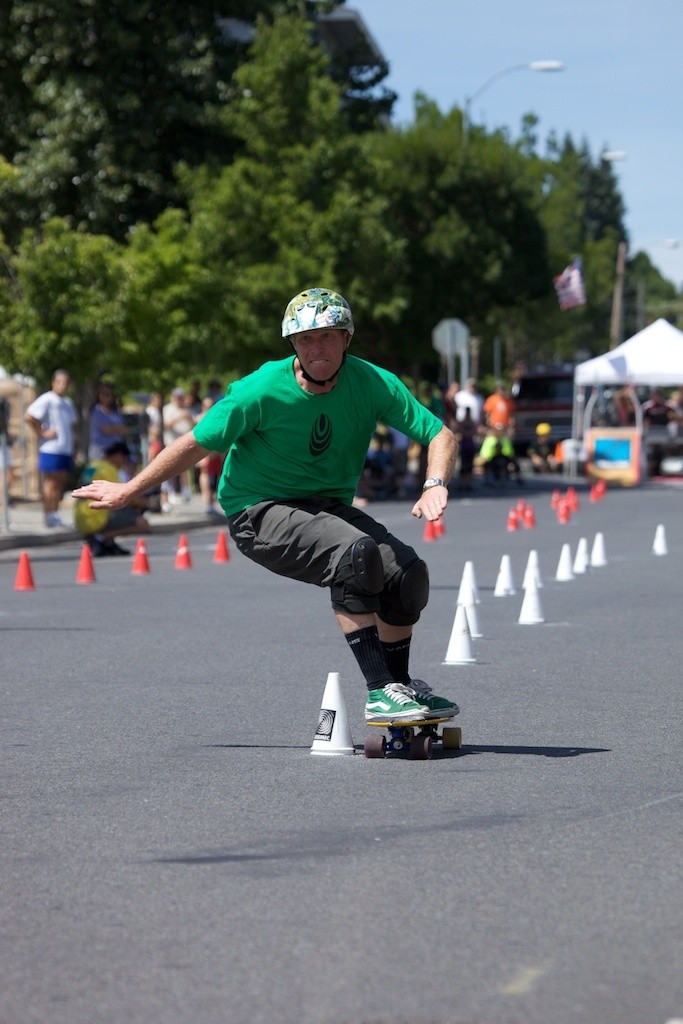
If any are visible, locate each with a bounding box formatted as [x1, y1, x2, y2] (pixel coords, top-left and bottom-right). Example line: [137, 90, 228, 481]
[364, 718, 462, 760]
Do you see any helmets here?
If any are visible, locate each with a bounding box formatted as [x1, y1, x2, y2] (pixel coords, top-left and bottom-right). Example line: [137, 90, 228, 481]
[282, 288, 354, 337]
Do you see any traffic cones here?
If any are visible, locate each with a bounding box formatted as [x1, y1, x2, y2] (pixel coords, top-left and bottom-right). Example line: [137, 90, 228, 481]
[173, 532, 193, 571]
[507, 507, 520, 533]
[430, 513, 445, 538]
[550, 478, 607, 523]
[76, 543, 98, 584]
[555, 541, 578, 581]
[590, 529, 610, 570]
[424, 517, 437, 546]
[491, 556, 520, 597]
[457, 585, 482, 641]
[12, 551, 35, 590]
[438, 603, 482, 668]
[132, 536, 152, 575]
[310, 671, 361, 756]
[517, 570, 547, 627]
[651, 523, 669, 556]
[210, 530, 229, 562]
[572, 536, 593, 574]
[455, 561, 482, 604]
[518, 548, 546, 588]
[517, 496, 531, 531]
[523, 504, 534, 529]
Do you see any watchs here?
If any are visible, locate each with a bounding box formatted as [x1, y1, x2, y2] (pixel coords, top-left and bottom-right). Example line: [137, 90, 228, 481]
[422, 477, 448, 491]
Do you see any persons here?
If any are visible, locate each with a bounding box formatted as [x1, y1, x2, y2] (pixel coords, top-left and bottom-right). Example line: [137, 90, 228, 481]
[140, 389, 225, 514]
[428, 378, 524, 491]
[23, 369, 82, 528]
[70, 440, 152, 556]
[527, 422, 563, 473]
[88, 383, 133, 459]
[0, 394, 16, 469]
[359, 421, 411, 498]
[72, 288, 462, 722]
[622, 391, 683, 475]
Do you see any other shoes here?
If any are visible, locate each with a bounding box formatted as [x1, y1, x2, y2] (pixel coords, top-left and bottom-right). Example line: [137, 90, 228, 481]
[44, 515, 62, 527]
[107, 544, 130, 555]
[86, 534, 108, 557]
[206, 506, 223, 517]
[163, 503, 172, 511]
[181, 491, 191, 501]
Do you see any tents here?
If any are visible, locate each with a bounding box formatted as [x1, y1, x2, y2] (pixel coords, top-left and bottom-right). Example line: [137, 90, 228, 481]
[572, 316, 682, 476]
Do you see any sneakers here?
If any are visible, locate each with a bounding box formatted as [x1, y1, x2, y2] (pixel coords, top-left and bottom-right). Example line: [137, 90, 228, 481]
[364, 683, 429, 717]
[405, 679, 459, 717]
